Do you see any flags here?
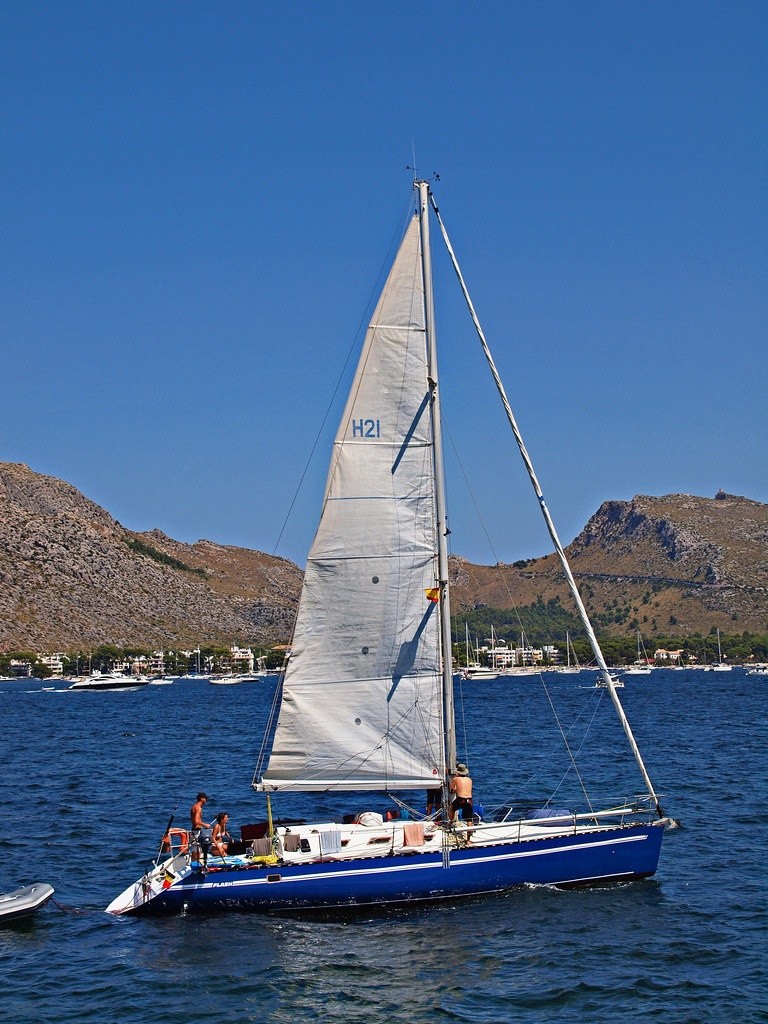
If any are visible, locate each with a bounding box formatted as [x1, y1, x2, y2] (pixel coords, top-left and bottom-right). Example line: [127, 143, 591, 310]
[424, 587, 440, 603]
[156, 867, 175, 890]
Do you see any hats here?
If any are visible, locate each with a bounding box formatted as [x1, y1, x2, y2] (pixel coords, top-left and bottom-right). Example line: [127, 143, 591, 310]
[454, 763, 468, 774]
[196, 792, 211, 799]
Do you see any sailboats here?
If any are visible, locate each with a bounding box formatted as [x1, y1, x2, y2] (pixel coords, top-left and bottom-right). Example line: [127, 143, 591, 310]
[96, 137, 687, 934]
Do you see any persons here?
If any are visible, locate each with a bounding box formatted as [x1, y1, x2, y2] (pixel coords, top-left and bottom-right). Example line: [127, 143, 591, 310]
[425, 766, 448, 821]
[446, 764, 475, 845]
[210, 812, 235, 856]
[190, 792, 212, 858]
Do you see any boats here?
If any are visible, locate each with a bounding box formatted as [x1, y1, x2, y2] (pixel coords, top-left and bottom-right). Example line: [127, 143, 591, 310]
[0, 882, 56, 923]
[459, 665, 652, 691]
[29, 672, 272, 693]
